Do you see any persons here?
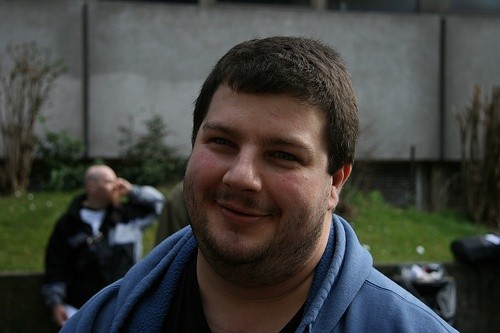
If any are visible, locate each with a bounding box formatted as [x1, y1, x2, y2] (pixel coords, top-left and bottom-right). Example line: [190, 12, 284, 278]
[42, 165, 192, 328]
[58, 35, 460, 333]
